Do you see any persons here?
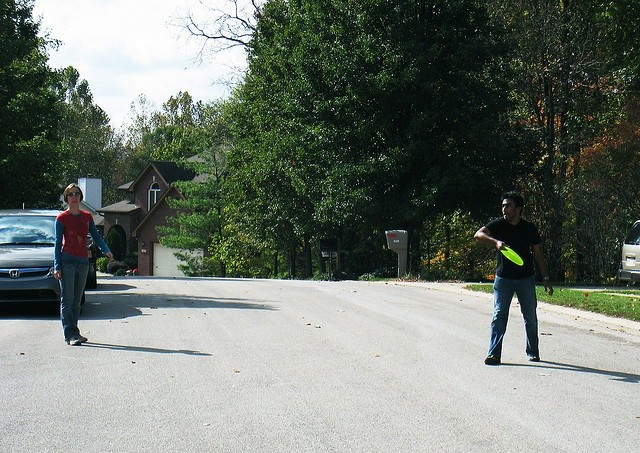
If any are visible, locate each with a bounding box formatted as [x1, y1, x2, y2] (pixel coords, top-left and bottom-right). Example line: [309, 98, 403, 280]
[54, 183, 113, 346]
[474, 193, 553, 364]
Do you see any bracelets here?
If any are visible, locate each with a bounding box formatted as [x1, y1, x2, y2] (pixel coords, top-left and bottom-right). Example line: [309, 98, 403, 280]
[543, 276, 551, 280]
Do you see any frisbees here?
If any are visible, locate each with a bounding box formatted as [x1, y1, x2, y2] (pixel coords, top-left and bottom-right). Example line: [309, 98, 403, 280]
[503, 248, 523, 266]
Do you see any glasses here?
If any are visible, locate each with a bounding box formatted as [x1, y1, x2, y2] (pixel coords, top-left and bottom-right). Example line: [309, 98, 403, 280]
[67, 190, 81, 196]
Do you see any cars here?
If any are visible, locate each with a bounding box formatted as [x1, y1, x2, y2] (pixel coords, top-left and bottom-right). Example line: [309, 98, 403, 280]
[1, 208, 85, 313]
[86, 231, 99, 287]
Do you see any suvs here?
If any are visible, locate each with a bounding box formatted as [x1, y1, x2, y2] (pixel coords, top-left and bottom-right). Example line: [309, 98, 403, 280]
[615, 219, 640, 284]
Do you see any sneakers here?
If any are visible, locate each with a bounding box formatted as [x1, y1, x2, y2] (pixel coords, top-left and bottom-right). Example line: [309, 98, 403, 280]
[484, 354, 501, 364]
[77, 333, 88, 341]
[67, 336, 81, 346]
[527, 352, 540, 362]
